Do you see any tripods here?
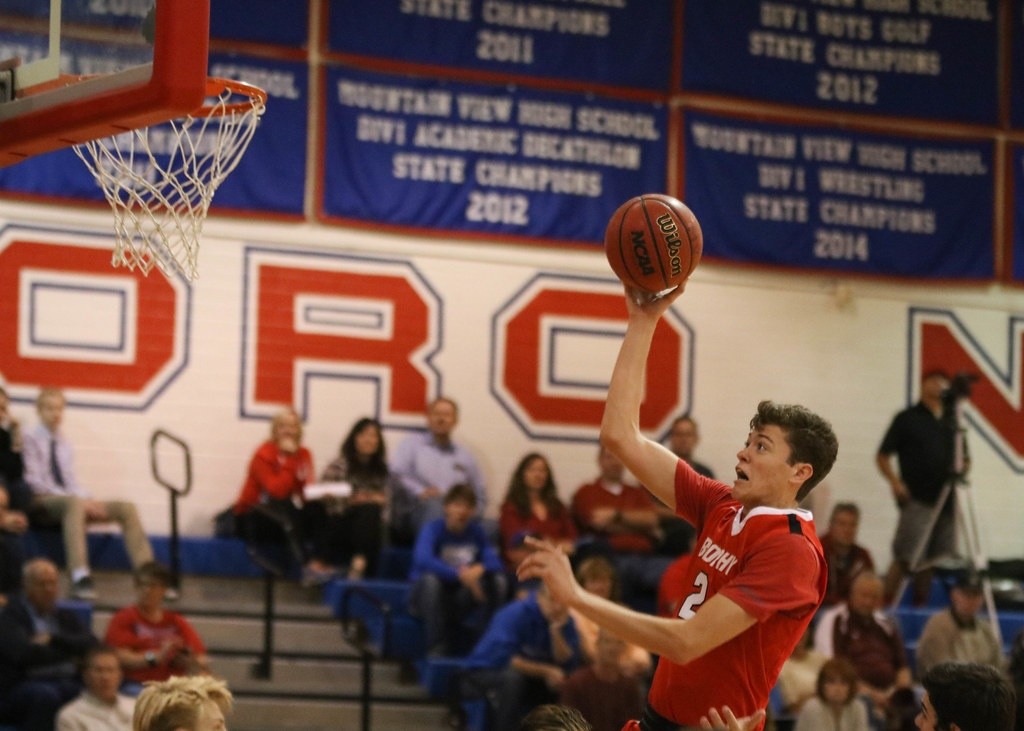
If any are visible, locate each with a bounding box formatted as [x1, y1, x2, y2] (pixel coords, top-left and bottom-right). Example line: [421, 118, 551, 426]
[884, 417, 1001, 647]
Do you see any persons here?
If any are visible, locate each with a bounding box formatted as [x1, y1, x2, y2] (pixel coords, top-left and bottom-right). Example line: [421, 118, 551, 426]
[53, 647, 136, 731]
[875, 369, 971, 609]
[106, 561, 211, 699]
[321, 417, 391, 579]
[915, 577, 1008, 675]
[131, 675, 234, 731]
[779, 620, 825, 714]
[699, 662, 1018, 731]
[0, 382, 97, 595]
[20, 388, 178, 599]
[0, 558, 91, 731]
[793, 658, 869, 731]
[386, 397, 716, 731]
[816, 571, 925, 724]
[233, 406, 337, 588]
[517, 275, 839, 731]
[817, 501, 873, 601]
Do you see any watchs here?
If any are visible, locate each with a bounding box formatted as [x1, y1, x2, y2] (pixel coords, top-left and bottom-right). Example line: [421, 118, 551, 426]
[145, 651, 156, 667]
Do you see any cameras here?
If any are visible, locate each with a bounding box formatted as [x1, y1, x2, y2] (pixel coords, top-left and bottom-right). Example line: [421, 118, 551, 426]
[939, 374, 972, 419]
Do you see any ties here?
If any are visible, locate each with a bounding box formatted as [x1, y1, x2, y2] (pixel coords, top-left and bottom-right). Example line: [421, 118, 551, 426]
[49, 441, 64, 486]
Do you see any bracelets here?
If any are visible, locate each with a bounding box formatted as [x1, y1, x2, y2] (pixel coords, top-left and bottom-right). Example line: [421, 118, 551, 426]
[614, 510, 622, 523]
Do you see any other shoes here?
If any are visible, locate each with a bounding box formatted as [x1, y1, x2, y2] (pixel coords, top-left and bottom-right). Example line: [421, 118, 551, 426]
[294, 558, 349, 588]
[66, 574, 97, 599]
[162, 587, 185, 603]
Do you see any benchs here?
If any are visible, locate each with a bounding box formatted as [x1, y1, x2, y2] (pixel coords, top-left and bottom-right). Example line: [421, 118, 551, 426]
[41, 505, 1024, 731]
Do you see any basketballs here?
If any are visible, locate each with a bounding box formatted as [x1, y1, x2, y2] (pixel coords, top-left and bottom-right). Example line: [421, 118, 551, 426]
[603, 193, 704, 295]
